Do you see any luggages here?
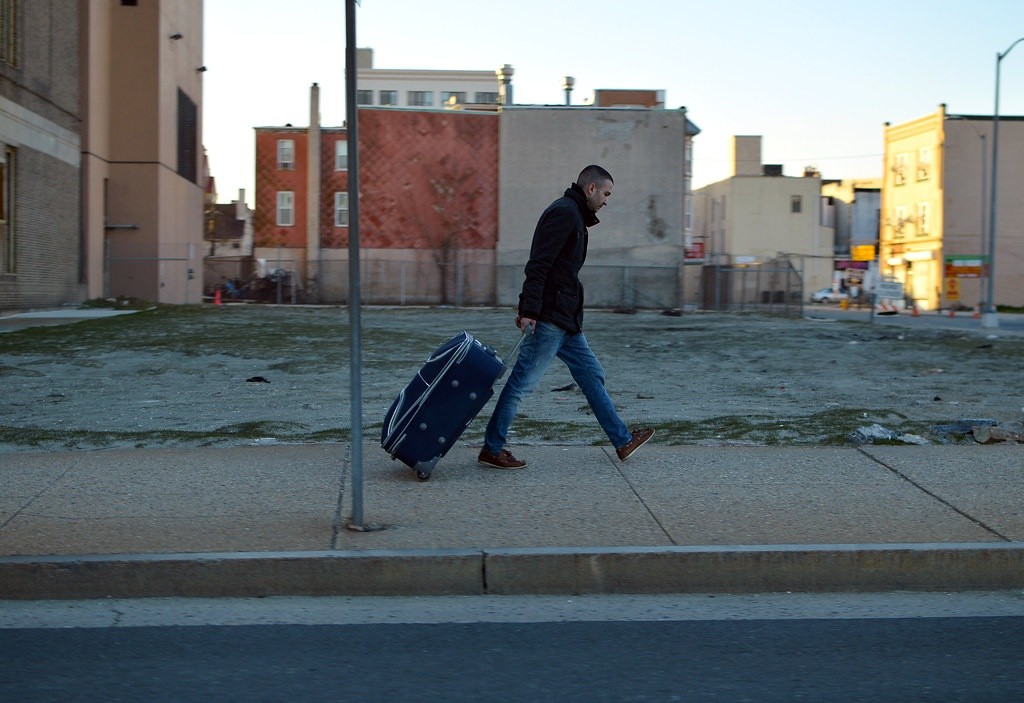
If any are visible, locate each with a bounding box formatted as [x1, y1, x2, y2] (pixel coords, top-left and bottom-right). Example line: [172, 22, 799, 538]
[380, 325, 533, 481]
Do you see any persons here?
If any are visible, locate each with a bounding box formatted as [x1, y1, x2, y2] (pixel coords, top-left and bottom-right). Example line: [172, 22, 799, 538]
[477, 165, 656, 470]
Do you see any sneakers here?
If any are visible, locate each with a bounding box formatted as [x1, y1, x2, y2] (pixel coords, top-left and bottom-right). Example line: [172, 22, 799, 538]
[478, 449, 528, 469]
[616, 428, 655, 461]
